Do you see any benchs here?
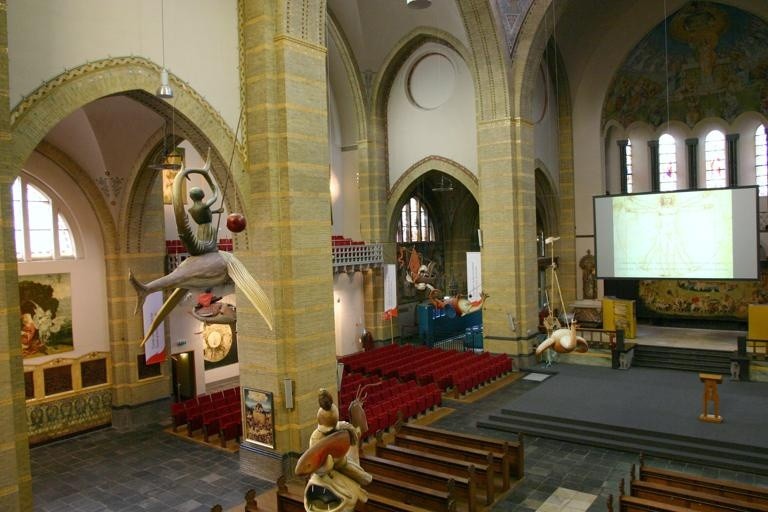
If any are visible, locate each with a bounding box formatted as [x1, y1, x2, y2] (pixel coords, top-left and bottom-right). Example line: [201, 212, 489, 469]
[212, 420, 525, 512]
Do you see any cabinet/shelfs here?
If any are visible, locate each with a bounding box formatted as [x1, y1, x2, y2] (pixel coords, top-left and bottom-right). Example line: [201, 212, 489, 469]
[607, 450, 765, 511]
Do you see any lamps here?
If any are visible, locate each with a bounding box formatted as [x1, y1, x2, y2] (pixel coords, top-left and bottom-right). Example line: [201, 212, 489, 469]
[155, 0, 174, 101]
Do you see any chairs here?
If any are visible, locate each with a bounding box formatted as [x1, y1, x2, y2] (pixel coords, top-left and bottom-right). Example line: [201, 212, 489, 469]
[168, 343, 516, 449]
